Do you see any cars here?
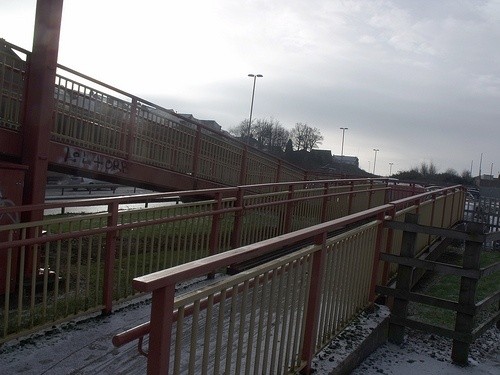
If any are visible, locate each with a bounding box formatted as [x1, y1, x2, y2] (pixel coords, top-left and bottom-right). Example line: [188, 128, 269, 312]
[70, 175, 117, 190]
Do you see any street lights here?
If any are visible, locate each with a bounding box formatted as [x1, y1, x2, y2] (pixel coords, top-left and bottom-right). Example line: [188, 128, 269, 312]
[371, 148, 379, 175]
[339, 127, 348, 173]
[245, 73, 263, 144]
[389, 163, 394, 176]
